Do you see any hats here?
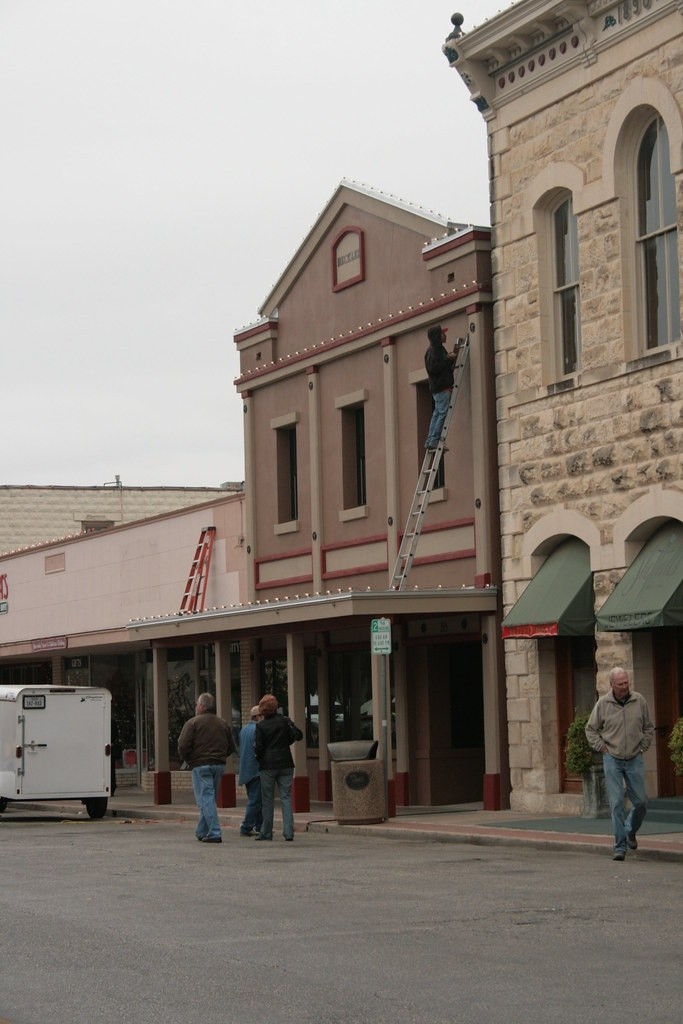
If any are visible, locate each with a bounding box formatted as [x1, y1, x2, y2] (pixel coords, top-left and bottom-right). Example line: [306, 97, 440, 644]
[250, 706, 260, 716]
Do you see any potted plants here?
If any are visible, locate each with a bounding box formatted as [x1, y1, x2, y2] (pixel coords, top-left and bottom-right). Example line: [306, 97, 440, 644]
[564, 706, 611, 820]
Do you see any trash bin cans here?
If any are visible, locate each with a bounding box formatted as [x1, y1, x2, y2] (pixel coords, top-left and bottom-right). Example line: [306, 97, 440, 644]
[327, 740, 386, 825]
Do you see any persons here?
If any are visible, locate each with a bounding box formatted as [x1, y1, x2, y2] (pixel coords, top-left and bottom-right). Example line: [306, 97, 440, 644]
[424, 326, 458, 450]
[238, 696, 304, 841]
[585, 666, 655, 861]
[178, 692, 236, 843]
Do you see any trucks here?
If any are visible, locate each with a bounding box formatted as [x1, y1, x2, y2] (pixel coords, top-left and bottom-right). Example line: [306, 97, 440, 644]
[0, 683, 115, 819]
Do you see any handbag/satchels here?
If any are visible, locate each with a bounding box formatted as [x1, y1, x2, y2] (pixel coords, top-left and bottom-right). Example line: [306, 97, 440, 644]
[285, 716, 299, 745]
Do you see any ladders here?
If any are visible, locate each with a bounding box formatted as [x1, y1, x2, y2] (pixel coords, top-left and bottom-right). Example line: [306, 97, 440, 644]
[388, 334, 470, 591]
[178, 526, 216, 615]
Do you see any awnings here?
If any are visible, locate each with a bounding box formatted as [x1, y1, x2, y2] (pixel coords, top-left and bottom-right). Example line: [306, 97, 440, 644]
[500, 537, 594, 639]
[595, 520, 683, 632]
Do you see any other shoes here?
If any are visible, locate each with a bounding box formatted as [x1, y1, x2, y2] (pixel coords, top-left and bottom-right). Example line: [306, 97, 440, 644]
[282, 832, 293, 841]
[613, 850, 625, 862]
[240, 830, 260, 837]
[255, 838, 272, 841]
[622, 821, 637, 849]
[202, 837, 222, 843]
